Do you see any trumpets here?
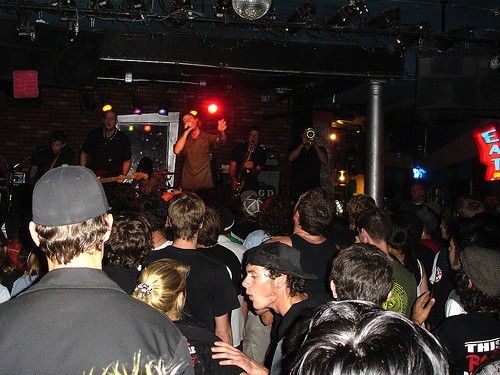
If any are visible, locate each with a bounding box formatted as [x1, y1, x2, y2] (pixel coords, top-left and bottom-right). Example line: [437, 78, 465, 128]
[304, 127, 319, 142]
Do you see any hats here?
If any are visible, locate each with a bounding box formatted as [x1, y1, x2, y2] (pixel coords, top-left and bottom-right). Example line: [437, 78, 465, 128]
[460, 246, 500, 297]
[32, 164, 112, 227]
[241, 190, 265, 218]
[248, 241, 320, 280]
[451, 211, 500, 250]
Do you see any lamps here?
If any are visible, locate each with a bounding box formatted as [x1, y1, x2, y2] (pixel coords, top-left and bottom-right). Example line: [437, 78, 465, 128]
[394, 19, 433, 49]
[16, 7, 37, 41]
[186, 94, 198, 116]
[282, 1, 317, 36]
[434, 25, 478, 51]
[368, 7, 402, 31]
[212, 0, 230, 18]
[86, 0, 115, 20]
[132, 94, 144, 115]
[231, 0, 272, 21]
[126, 0, 146, 20]
[99, 89, 113, 111]
[57, 0, 80, 20]
[167, 0, 191, 28]
[325, 0, 368, 31]
[159, 93, 171, 115]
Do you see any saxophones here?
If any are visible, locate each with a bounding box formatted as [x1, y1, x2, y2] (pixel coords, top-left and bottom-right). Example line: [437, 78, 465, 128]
[233, 142, 257, 197]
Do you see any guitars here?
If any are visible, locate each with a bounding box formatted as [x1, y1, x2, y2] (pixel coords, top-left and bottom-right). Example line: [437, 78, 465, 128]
[98, 172, 149, 183]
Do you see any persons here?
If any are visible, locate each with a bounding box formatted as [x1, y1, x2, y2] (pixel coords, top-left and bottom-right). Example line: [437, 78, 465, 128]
[0, 109, 500, 375]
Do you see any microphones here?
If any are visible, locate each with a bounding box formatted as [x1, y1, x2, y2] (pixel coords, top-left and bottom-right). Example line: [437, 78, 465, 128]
[251, 140, 255, 147]
[184, 125, 193, 131]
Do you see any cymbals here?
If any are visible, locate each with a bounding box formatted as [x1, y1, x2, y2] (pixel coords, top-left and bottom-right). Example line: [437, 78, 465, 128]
[152, 171, 180, 175]
